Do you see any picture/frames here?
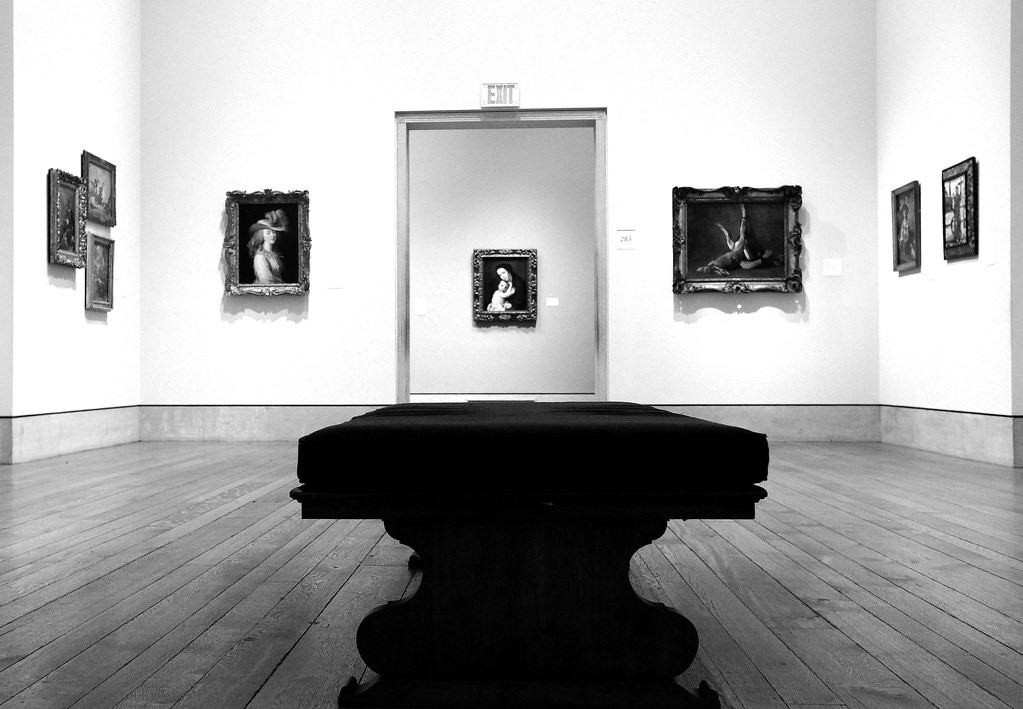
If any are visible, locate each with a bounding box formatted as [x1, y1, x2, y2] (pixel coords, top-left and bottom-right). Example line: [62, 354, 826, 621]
[82, 150, 116, 227]
[941, 156, 979, 260]
[472, 248, 538, 322]
[673, 185, 803, 294]
[891, 181, 921, 272]
[48, 168, 89, 269]
[225, 189, 312, 296]
[85, 231, 115, 312]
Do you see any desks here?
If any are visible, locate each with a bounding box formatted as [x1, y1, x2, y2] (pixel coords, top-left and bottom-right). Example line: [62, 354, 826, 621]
[289, 401, 770, 708]
